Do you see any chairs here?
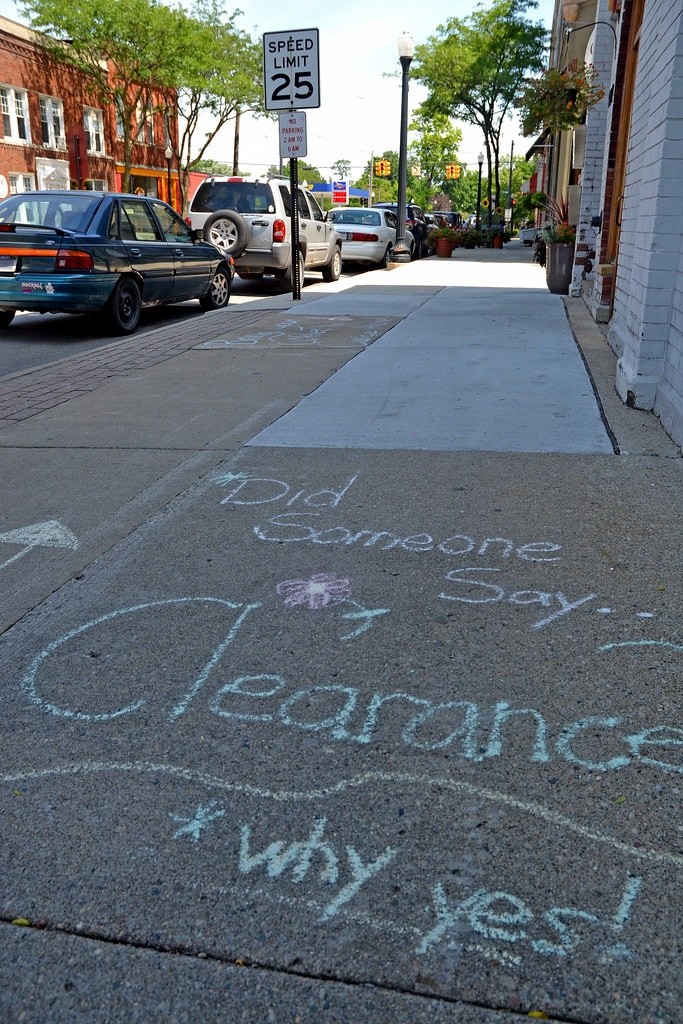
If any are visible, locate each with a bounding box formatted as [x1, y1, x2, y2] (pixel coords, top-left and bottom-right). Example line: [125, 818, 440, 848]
[61, 210, 82, 229]
[236, 199, 250, 213]
[111, 221, 136, 241]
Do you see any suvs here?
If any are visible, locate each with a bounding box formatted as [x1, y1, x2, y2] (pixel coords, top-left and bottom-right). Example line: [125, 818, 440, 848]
[371, 201, 434, 261]
[182, 173, 342, 293]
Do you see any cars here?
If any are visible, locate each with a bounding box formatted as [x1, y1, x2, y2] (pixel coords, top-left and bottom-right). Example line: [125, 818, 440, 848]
[423, 211, 481, 248]
[0, 189, 235, 338]
[328, 206, 416, 269]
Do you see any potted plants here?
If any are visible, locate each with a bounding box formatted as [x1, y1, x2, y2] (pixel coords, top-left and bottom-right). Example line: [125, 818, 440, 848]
[459, 228, 479, 248]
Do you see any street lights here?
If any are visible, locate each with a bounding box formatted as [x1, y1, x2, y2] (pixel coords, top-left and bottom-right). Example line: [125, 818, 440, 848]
[165, 146, 172, 206]
[476, 152, 484, 231]
[388, 30, 412, 264]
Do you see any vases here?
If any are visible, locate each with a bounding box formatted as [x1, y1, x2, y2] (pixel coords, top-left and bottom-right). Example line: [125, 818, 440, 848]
[545, 243, 575, 295]
[493, 236, 502, 248]
[434, 237, 453, 257]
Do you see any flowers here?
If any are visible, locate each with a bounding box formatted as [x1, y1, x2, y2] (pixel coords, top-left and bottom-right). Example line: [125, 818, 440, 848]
[513, 56, 605, 136]
[486, 224, 502, 241]
[538, 222, 577, 244]
[427, 224, 461, 250]
